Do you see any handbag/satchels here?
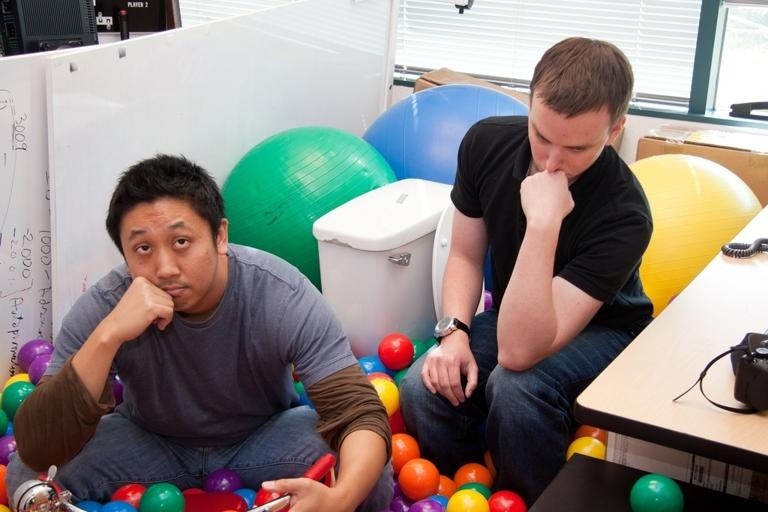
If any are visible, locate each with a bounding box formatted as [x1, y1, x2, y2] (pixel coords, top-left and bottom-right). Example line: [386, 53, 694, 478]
[731, 328, 768, 413]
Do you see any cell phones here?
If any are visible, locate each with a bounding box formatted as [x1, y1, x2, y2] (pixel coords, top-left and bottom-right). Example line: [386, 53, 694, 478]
[246, 493, 291, 512]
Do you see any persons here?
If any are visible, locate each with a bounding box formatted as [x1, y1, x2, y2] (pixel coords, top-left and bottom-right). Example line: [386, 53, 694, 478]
[399, 35, 656, 510]
[12, 153, 393, 512]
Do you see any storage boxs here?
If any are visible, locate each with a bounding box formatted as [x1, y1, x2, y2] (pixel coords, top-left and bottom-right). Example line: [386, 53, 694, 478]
[635, 123, 768, 210]
[413, 67, 531, 108]
[606, 431, 754, 502]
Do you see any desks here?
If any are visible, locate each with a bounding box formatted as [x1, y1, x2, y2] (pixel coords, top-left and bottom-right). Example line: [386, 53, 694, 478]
[574, 203, 768, 473]
[527, 453, 767, 512]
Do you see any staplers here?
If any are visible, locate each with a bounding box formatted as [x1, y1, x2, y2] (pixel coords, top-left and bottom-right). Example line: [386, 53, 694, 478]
[729, 102, 768, 120]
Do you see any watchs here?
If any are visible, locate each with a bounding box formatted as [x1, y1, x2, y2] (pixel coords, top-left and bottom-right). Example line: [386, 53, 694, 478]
[433, 316, 471, 347]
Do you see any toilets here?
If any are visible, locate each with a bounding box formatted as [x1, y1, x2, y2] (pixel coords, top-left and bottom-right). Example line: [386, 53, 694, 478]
[312, 178, 486, 359]
